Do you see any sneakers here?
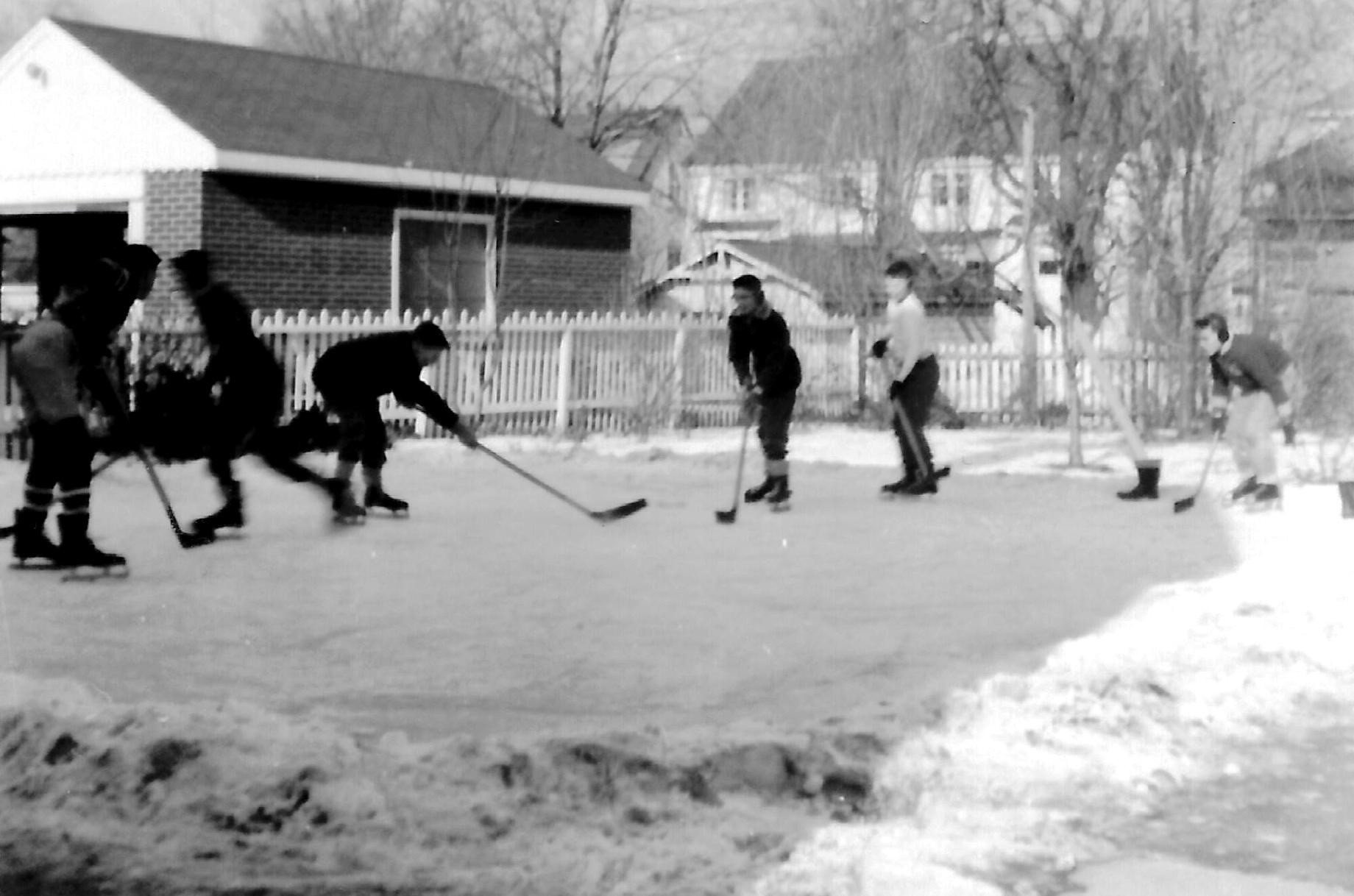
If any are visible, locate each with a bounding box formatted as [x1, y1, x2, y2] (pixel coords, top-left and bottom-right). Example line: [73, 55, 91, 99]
[12, 539, 60, 557]
[53, 540, 127, 566]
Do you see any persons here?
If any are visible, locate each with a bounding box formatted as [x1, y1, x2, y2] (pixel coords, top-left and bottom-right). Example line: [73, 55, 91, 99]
[728, 274, 801, 504]
[170, 249, 349, 530]
[873, 262, 940, 495]
[312, 320, 477, 516]
[13, 244, 163, 568]
[1194, 313, 1302, 501]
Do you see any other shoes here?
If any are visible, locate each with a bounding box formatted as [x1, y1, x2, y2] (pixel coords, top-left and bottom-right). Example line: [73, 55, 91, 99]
[330, 488, 369, 517]
[192, 480, 245, 529]
[745, 474, 776, 502]
[1231, 476, 1261, 500]
[1255, 480, 1279, 501]
[763, 475, 791, 502]
[882, 460, 919, 492]
[364, 484, 409, 510]
[901, 463, 938, 492]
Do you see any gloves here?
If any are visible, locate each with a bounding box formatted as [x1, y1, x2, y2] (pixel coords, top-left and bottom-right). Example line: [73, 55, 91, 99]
[872, 340, 890, 358]
[889, 380, 903, 402]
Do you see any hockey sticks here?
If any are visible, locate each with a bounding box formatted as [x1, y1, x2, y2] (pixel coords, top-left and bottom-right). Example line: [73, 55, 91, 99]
[134, 443, 217, 549]
[880, 357, 951, 483]
[413, 405, 649, 524]
[0, 456, 119, 539]
[715, 427, 749, 524]
[1173, 401, 1236, 516]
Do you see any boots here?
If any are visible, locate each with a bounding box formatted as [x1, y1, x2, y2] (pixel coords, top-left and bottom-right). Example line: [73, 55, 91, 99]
[1117, 458, 1160, 500]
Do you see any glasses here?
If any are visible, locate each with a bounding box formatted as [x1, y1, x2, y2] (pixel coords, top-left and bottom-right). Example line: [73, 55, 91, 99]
[732, 293, 753, 299]
